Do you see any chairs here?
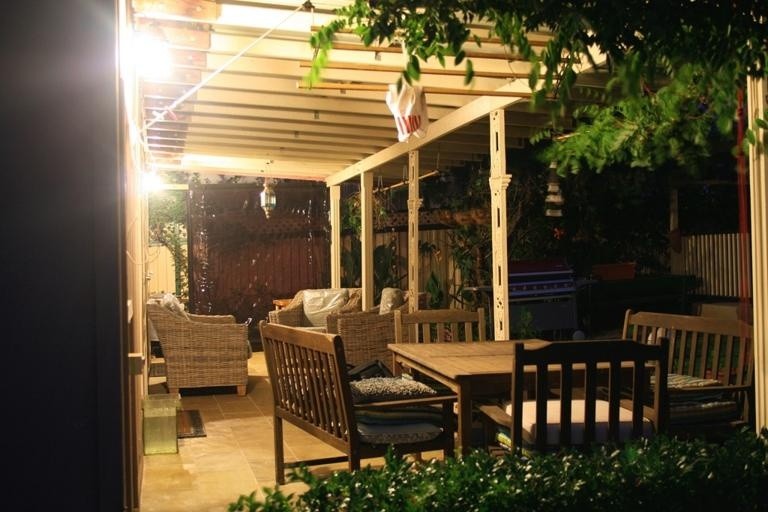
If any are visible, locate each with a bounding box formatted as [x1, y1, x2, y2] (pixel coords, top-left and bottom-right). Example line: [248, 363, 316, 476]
[146, 285, 432, 397]
[391, 308, 503, 425]
[476, 335, 674, 464]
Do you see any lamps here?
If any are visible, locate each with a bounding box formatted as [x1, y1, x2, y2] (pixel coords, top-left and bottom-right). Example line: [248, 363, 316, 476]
[259, 178, 277, 220]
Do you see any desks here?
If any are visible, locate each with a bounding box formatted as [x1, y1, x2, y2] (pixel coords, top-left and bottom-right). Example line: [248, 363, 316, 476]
[382, 338, 665, 459]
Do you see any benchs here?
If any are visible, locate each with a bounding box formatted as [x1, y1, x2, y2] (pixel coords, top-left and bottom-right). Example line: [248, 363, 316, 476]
[260, 318, 459, 488]
[606, 308, 754, 444]
